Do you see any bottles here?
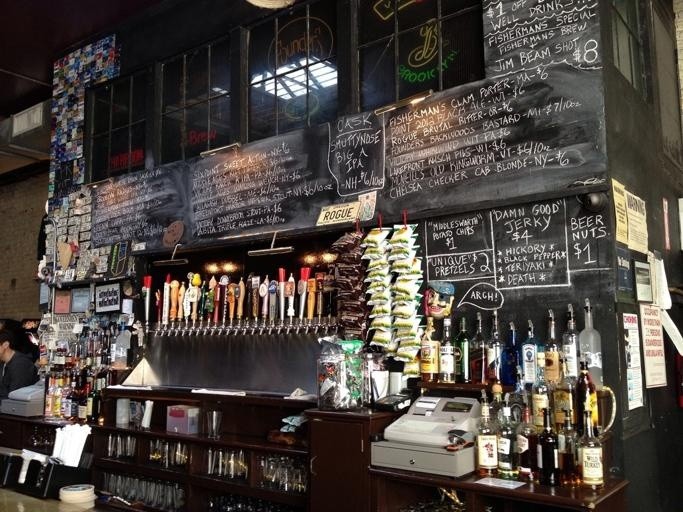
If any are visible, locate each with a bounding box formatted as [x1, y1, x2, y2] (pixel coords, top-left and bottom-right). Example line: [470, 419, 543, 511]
[415, 297, 618, 499]
[43, 318, 128, 422]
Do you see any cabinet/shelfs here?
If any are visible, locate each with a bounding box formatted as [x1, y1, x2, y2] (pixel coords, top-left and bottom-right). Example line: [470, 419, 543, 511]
[91, 390, 394, 512]
[370, 463, 628, 512]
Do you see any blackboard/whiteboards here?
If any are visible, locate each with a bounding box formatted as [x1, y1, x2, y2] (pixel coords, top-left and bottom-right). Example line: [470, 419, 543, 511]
[361, 193, 625, 478]
[91, 1, 610, 282]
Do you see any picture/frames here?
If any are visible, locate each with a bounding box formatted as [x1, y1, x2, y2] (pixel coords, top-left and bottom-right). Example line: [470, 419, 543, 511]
[94, 282, 122, 314]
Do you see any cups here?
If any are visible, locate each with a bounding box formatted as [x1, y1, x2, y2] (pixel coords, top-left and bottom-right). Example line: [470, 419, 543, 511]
[105, 473, 186, 512]
[149, 439, 188, 469]
[104, 433, 135, 461]
[205, 447, 246, 479]
[209, 497, 280, 512]
[258, 456, 307, 493]
[207, 410, 222, 440]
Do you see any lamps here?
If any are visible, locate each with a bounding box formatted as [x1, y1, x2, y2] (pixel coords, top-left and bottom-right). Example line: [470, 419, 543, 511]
[374, 89, 432, 116]
[248, 230, 295, 256]
[79, 178, 112, 188]
[152, 244, 188, 266]
[199, 143, 241, 158]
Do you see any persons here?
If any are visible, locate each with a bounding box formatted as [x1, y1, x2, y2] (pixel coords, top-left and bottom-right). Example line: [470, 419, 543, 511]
[0, 326, 41, 398]
